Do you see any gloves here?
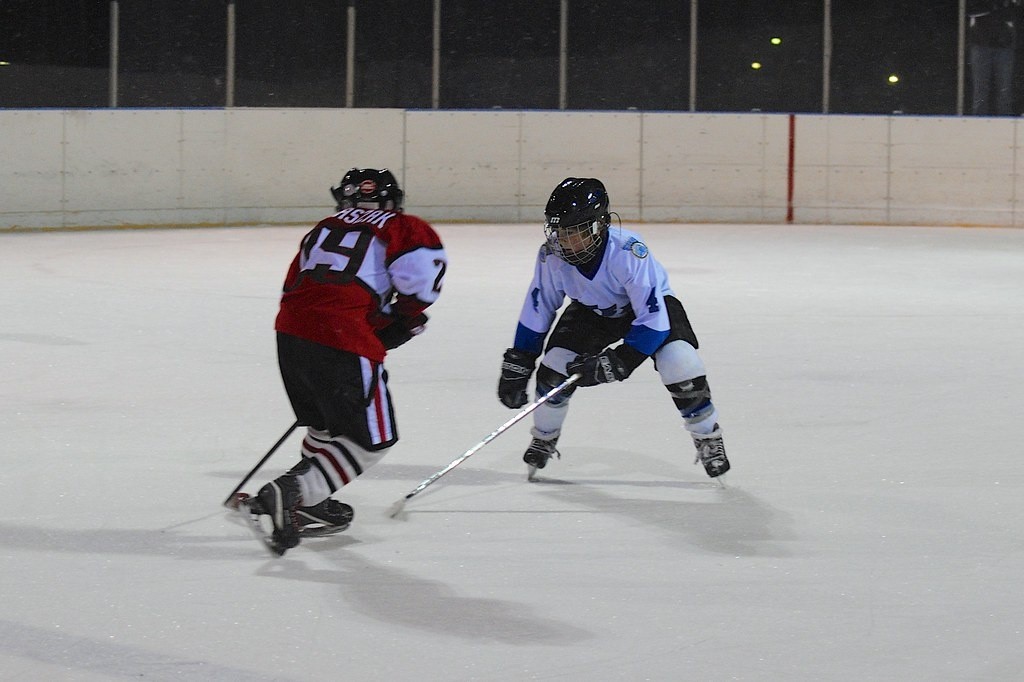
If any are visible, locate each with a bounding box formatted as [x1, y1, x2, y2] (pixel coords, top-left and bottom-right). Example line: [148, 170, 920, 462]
[497, 349, 536, 409]
[565, 347, 632, 389]
[373, 310, 428, 349]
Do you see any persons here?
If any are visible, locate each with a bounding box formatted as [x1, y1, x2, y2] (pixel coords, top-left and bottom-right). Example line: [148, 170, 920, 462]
[498, 177, 730, 489]
[239, 166, 448, 557]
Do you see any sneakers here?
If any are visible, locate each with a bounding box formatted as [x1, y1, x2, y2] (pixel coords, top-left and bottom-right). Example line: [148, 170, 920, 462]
[691, 424, 731, 487]
[238, 472, 301, 558]
[296, 496, 354, 537]
[523, 427, 561, 480]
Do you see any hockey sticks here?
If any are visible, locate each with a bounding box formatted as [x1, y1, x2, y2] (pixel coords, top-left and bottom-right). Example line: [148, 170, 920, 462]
[383, 372, 583, 520]
[222, 419, 301, 510]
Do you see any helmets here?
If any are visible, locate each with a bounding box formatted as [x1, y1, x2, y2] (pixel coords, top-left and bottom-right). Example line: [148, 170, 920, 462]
[330, 168, 404, 210]
[543, 176, 612, 265]
